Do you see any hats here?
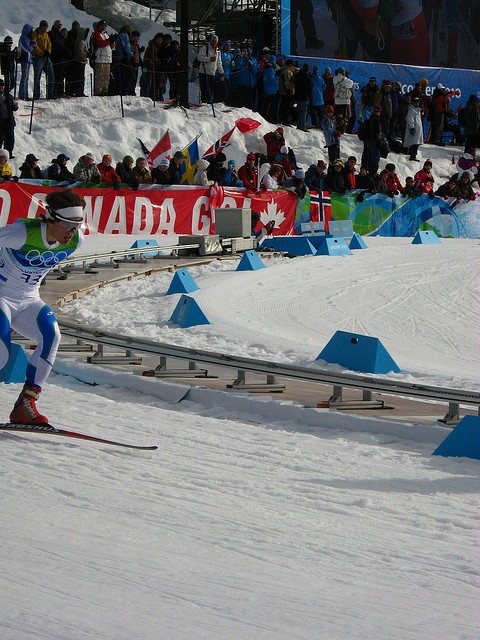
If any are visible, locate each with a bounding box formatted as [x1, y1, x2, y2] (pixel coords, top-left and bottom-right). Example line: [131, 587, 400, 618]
[424, 159, 432, 168]
[40, 21, 48, 28]
[0, 149, 9, 160]
[196, 159, 210, 170]
[247, 152, 256, 160]
[54, 20, 62, 27]
[339, 67, 346, 74]
[0, 79, 5, 84]
[420, 78, 428, 85]
[386, 164, 395, 172]
[4, 36, 13, 43]
[269, 55, 276, 62]
[26, 154, 39, 162]
[222, 40, 231, 50]
[406, 177, 413, 184]
[313, 65, 319, 72]
[286, 59, 294, 67]
[278, 128, 283, 133]
[384, 80, 391, 85]
[317, 160, 327, 169]
[370, 77, 376, 82]
[332, 159, 343, 166]
[159, 158, 169, 167]
[57, 154, 70, 161]
[295, 170, 305, 180]
[210, 35, 218, 43]
[85, 153, 94, 160]
[123, 156, 133, 166]
[436, 83, 445, 89]
[449, 173, 458, 184]
[326, 105, 334, 113]
[98, 20, 107, 26]
[280, 146, 289, 154]
[216, 152, 226, 162]
[461, 172, 470, 178]
[174, 151, 184, 157]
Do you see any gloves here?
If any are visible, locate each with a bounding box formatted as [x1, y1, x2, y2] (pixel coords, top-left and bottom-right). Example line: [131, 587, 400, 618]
[391, 190, 399, 195]
[402, 191, 406, 198]
[442, 194, 449, 200]
[410, 128, 416, 136]
[314, 187, 322, 194]
[357, 191, 365, 202]
[210, 57, 216, 62]
[428, 192, 434, 200]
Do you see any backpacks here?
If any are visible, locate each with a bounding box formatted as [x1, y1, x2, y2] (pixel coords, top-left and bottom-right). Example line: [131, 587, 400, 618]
[358, 117, 377, 141]
[193, 45, 208, 69]
[264, 132, 281, 158]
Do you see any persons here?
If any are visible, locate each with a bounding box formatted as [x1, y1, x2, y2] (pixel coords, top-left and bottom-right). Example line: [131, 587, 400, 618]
[223, 160, 241, 187]
[284, 169, 306, 192]
[18, 154, 44, 179]
[193, 160, 215, 188]
[150, 158, 177, 185]
[323, 104, 342, 163]
[455, 172, 476, 201]
[306, 160, 328, 197]
[404, 177, 424, 199]
[131, 157, 157, 187]
[413, 158, 436, 200]
[96, 154, 122, 190]
[0, 149, 12, 184]
[262, 163, 285, 191]
[342, 156, 357, 191]
[0, 75, 18, 155]
[273, 144, 295, 178]
[371, 168, 394, 198]
[435, 172, 462, 203]
[48, 154, 76, 184]
[326, 158, 348, 195]
[355, 164, 377, 202]
[73, 152, 102, 185]
[251, 211, 275, 237]
[0, 191, 86, 428]
[168, 151, 188, 185]
[206, 152, 228, 184]
[116, 155, 140, 191]
[291, 0, 480, 67]
[263, 127, 286, 163]
[380, 164, 405, 195]
[237, 152, 266, 197]
[362, 106, 388, 174]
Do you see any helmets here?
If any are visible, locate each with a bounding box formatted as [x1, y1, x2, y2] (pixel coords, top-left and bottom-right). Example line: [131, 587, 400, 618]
[102, 155, 112, 163]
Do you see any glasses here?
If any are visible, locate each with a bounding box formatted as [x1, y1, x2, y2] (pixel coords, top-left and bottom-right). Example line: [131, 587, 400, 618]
[47, 206, 84, 231]
[349, 157, 356, 161]
[374, 108, 382, 112]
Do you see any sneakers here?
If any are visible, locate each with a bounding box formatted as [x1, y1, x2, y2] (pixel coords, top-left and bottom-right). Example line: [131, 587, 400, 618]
[9, 382, 48, 424]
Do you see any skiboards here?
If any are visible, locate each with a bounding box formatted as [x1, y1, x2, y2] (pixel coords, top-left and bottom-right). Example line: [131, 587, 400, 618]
[0, 423, 158, 450]
